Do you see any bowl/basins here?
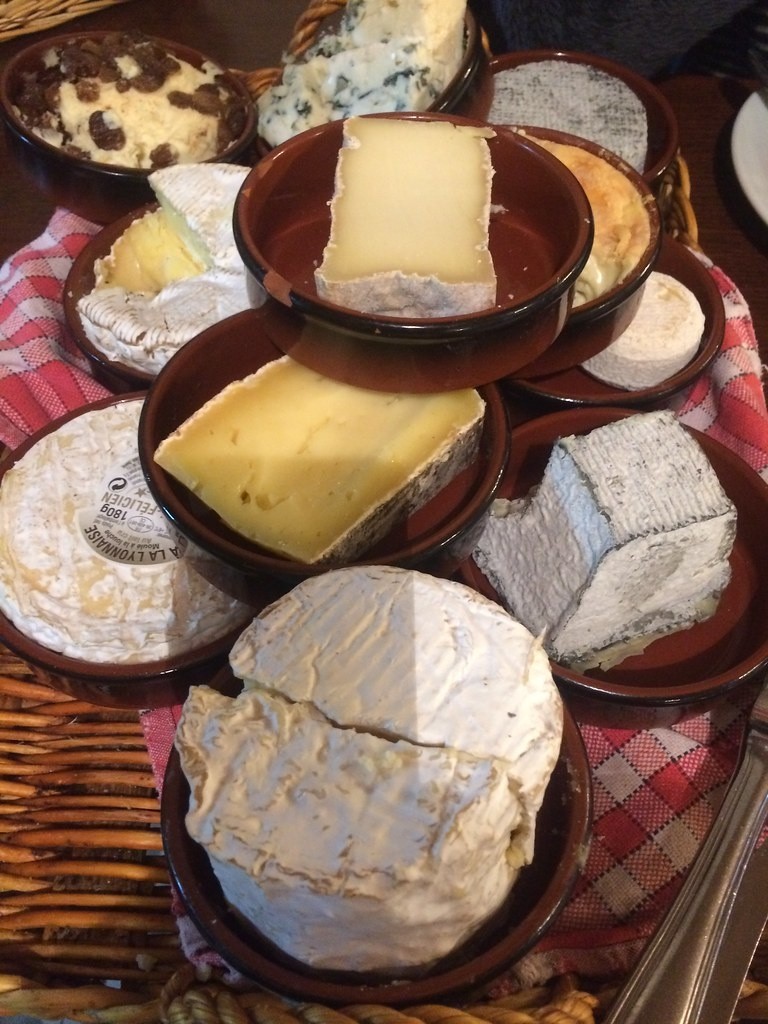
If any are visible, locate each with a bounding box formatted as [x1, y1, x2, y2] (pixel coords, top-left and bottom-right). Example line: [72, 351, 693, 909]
[0, 0, 767, 1003]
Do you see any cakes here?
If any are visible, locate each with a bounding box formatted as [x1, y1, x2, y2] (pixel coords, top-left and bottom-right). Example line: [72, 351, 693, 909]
[0, 2, 739, 962]
